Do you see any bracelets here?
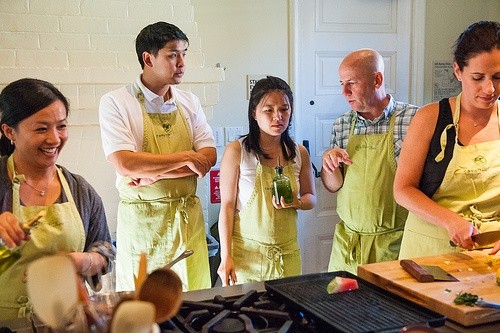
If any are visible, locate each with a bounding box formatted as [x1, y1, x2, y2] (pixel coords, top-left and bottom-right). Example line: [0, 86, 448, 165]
[294, 198, 304, 211]
[79, 250, 95, 276]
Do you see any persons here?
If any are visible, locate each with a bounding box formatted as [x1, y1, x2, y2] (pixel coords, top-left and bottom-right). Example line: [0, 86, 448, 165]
[217, 75, 318, 284]
[390, 20, 500, 260]
[318, 48, 423, 277]
[1, 77, 118, 322]
[100, 21, 219, 292]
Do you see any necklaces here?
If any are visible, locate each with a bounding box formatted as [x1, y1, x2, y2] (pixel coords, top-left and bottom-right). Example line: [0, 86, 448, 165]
[461, 108, 496, 130]
[13, 166, 59, 198]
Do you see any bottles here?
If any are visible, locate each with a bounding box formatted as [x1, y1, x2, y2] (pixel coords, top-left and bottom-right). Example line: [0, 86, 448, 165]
[271, 166, 294, 205]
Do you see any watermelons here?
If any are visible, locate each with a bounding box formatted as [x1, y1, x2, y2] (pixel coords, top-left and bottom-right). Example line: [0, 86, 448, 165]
[326, 276, 358, 294]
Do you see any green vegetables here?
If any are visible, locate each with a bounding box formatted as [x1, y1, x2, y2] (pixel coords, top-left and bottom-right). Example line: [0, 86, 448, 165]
[446, 288, 478, 307]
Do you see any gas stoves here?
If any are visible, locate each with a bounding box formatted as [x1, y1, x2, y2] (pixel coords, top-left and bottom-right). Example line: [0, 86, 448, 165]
[154, 288, 340, 333]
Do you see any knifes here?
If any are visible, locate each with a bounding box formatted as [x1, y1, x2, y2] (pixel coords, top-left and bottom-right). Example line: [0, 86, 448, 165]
[450, 230, 500, 247]
[400, 260, 459, 282]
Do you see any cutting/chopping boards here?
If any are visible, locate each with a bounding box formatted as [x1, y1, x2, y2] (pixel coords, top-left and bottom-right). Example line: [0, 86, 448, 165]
[357, 249, 500, 327]
